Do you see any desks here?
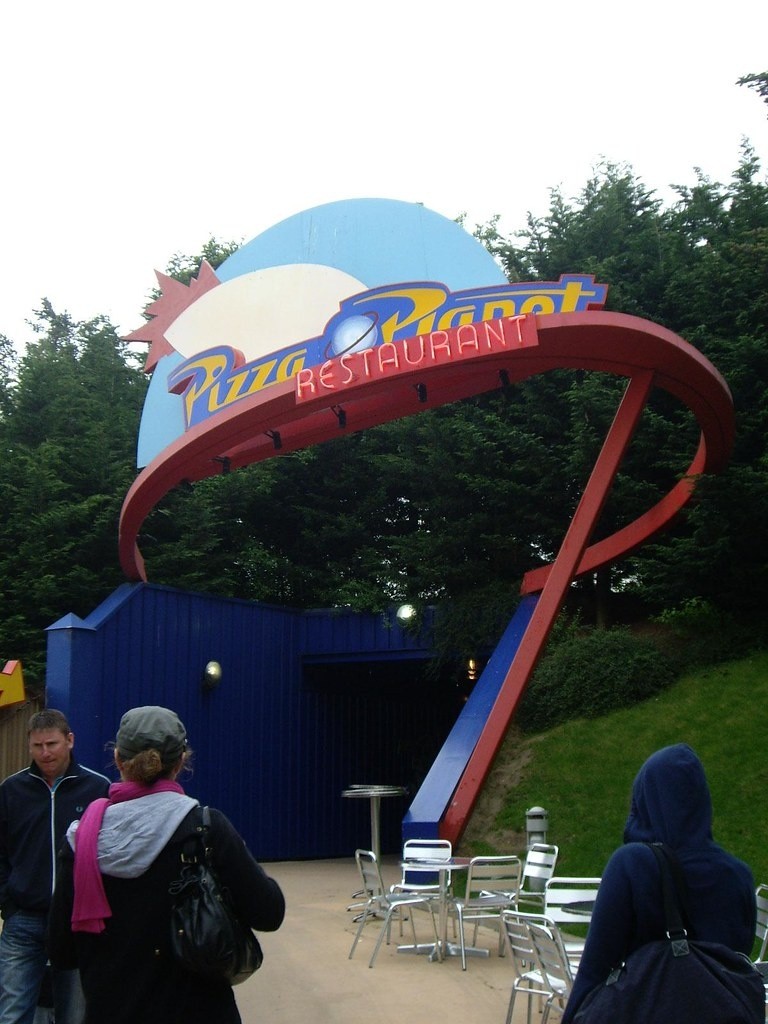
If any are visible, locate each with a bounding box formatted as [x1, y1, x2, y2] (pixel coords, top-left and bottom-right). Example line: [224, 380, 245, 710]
[341, 781, 412, 903]
[395, 856, 491, 964]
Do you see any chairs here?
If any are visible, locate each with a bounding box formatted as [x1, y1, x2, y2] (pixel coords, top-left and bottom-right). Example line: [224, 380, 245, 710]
[471, 841, 561, 959]
[529, 920, 577, 1024]
[542, 875, 604, 1014]
[385, 838, 453, 951]
[452, 852, 528, 971]
[347, 845, 442, 971]
[748, 884, 768, 964]
[503, 906, 589, 1024]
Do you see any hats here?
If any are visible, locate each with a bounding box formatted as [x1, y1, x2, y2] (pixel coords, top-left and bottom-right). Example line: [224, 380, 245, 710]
[116, 705, 188, 762]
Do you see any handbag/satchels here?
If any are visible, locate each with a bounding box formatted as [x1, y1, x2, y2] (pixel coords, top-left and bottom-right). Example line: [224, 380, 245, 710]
[572, 844, 767, 1024]
[165, 805, 265, 989]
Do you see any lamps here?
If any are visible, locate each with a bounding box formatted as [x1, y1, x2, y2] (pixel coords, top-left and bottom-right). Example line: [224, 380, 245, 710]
[521, 804, 556, 891]
[203, 656, 226, 691]
[395, 605, 425, 631]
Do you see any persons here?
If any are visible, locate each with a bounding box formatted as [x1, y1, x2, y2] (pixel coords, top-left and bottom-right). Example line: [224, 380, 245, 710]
[562, 743, 757, 1024]
[0, 705, 285, 1024]
